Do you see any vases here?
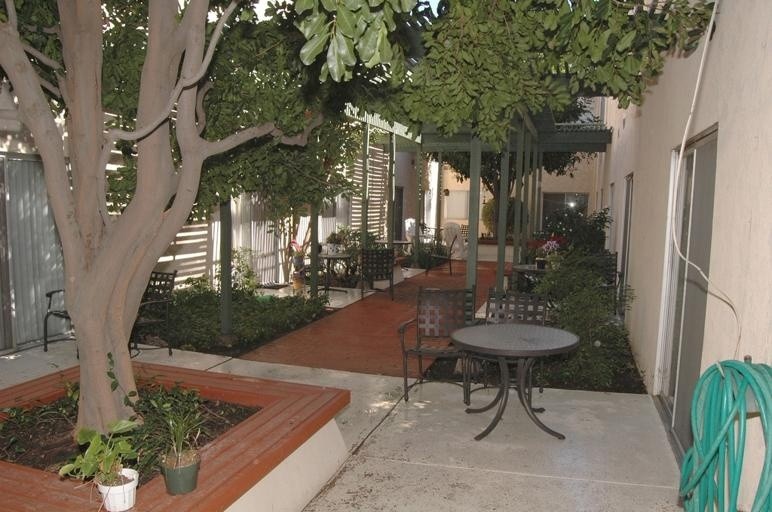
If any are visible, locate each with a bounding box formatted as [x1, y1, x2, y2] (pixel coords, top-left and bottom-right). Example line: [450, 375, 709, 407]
[292, 256, 304, 270]
[293, 280, 304, 289]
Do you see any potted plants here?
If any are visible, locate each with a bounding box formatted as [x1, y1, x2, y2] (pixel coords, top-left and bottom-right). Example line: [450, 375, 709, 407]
[326, 232, 343, 255]
[120, 384, 232, 498]
[59, 417, 140, 512]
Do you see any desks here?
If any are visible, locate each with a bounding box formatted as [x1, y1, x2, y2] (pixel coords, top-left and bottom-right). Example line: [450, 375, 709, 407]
[450, 324, 578, 441]
[307, 253, 351, 296]
[512, 263, 551, 291]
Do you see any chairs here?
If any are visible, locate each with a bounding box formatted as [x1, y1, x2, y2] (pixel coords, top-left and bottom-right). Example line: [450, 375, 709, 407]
[41, 288, 81, 360]
[396, 284, 478, 406]
[126, 269, 177, 356]
[466, 286, 555, 408]
[357, 218, 472, 302]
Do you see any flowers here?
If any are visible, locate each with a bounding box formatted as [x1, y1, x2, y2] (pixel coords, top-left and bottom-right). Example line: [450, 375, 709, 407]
[288, 241, 306, 257]
[291, 270, 306, 281]
